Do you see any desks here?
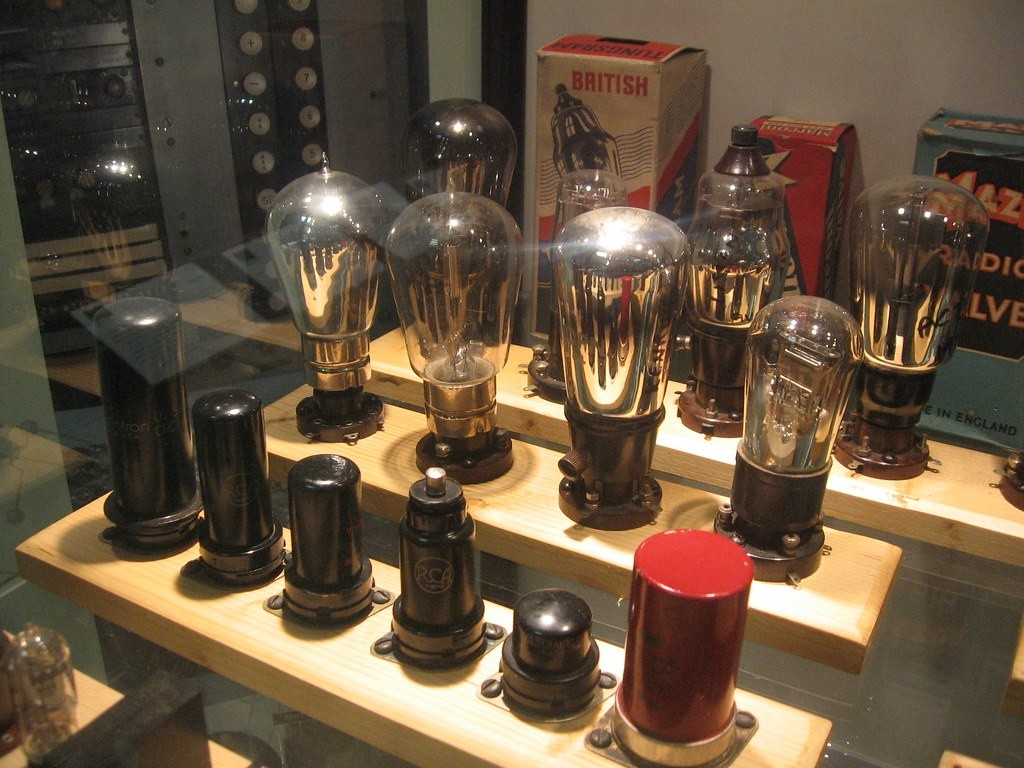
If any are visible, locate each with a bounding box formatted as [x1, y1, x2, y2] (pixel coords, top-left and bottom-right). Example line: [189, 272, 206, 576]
[366, 319, 1024, 687]
[1, 627, 252, 768]
[256, 383, 901, 673]
[17, 480, 834, 768]
